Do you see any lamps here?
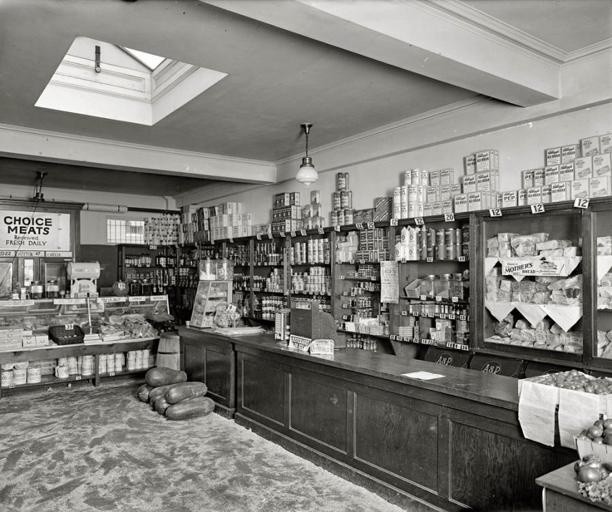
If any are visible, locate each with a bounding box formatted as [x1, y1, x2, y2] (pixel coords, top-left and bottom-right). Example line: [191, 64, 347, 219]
[296, 122, 318, 187]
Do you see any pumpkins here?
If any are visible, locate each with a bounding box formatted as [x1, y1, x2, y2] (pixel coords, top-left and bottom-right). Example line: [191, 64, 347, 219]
[136, 366, 217, 421]
